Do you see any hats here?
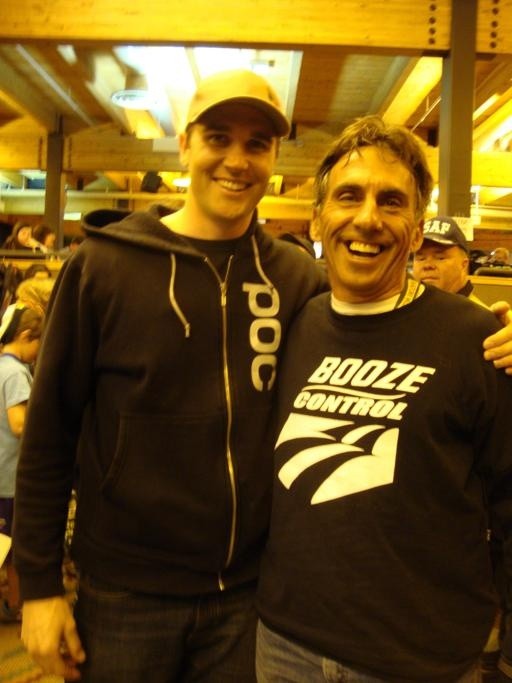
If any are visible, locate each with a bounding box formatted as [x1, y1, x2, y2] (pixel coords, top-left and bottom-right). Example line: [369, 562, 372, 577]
[182, 73, 292, 138]
[419, 218, 471, 251]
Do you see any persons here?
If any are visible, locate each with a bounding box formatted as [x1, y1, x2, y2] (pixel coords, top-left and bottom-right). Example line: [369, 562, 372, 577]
[1, 215, 81, 535]
[415, 216, 512, 307]
[257, 112, 512, 680]
[13, 67, 512, 682]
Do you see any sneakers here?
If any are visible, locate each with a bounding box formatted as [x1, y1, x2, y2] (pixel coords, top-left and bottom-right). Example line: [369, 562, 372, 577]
[1, 599, 26, 622]
